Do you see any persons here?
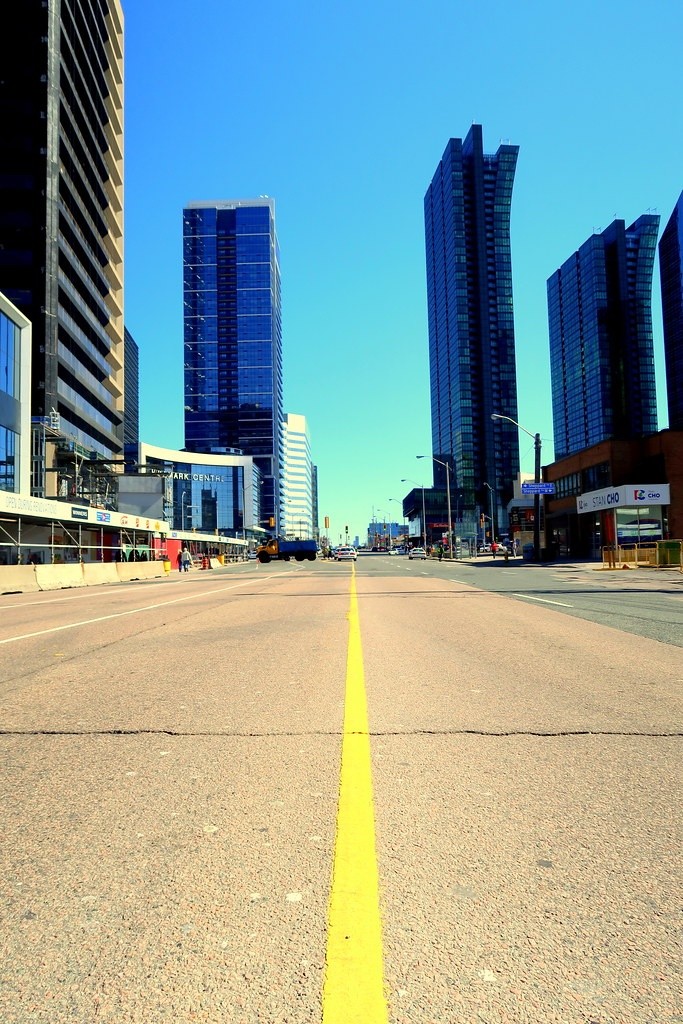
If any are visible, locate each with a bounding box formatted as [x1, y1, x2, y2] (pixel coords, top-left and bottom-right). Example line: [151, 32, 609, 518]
[183, 548, 193, 572]
[177, 550, 183, 571]
[141, 551, 148, 561]
[115, 546, 128, 562]
[511, 539, 517, 558]
[129, 544, 140, 562]
[489, 540, 498, 558]
[402, 544, 456, 563]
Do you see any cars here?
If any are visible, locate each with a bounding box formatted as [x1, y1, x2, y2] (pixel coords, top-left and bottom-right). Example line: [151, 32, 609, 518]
[338, 547, 357, 562]
[334, 548, 341, 561]
[479, 543, 507, 553]
[408, 547, 426, 561]
[388, 548, 405, 555]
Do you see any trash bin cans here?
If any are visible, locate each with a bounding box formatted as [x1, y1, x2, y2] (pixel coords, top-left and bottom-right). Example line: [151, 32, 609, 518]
[522, 544, 534, 561]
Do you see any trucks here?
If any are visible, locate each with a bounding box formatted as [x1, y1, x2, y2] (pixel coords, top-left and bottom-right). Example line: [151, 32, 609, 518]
[256, 537, 317, 564]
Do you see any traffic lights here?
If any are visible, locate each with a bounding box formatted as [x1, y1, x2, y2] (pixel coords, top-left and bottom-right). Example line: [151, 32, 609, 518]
[481, 513, 485, 522]
[345, 526, 349, 534]
[384, 524, 386, 530]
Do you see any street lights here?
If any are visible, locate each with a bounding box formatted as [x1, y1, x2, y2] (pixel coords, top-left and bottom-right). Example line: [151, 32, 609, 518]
[416, 455, 454, 559]
[401, 478, 427, 551]
[181, 491, 186, 531]
[241, 481, 264, 540]
[389, 499, 406, 547]
[484, 482, 494, 543]
[377, 509, 392, 550]
[490, 414, 542, 561]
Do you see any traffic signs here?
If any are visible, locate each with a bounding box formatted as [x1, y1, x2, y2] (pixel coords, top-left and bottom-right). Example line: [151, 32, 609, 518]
[522, 482, 555, 488]
[522, 488, 556, 494]
